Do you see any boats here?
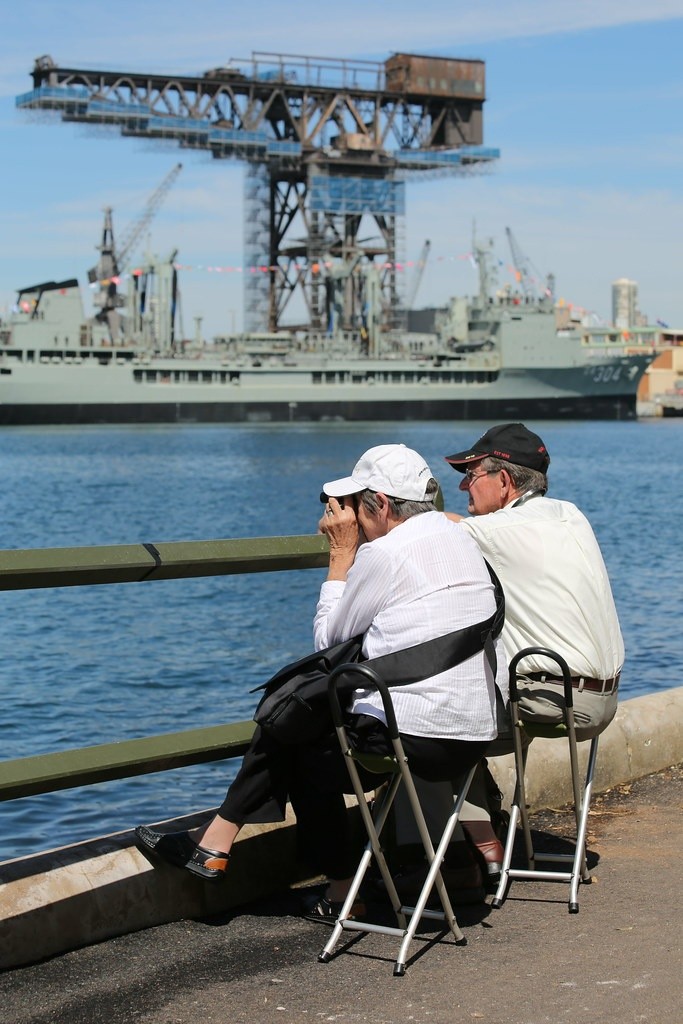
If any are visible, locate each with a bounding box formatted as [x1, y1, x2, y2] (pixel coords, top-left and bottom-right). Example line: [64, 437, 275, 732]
[0, 246, 664, 423]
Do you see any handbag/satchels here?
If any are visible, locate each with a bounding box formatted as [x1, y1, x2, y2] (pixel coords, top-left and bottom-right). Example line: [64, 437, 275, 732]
[249, 633, 365, 736]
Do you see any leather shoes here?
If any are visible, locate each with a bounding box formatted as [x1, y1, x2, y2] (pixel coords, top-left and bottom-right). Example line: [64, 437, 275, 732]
[134, 823, 231, 884]
[294, 895, 369, 928]
[474, 838, 504, 883]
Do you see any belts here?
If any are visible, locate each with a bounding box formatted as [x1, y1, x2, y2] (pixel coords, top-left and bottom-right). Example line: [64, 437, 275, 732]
[517, 668, 622, 692]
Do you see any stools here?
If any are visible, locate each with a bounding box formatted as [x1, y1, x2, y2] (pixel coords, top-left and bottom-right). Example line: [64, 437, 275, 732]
[319, 648, 600, 976]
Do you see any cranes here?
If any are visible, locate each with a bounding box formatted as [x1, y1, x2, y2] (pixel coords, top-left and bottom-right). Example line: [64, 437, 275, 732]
[473, 215, 546, 305]
[400, 240, 432, 330]
[99, 161, 182, 347]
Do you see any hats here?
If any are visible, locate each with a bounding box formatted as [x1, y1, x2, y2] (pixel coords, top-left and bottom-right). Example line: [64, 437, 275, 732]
[323, 443, 440, 502]
[443, 422, 550, 475]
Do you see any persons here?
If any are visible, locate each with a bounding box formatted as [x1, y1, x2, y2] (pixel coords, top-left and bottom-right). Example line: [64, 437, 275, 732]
[446, 423, 624, 875]
[135, 443, 499, 925]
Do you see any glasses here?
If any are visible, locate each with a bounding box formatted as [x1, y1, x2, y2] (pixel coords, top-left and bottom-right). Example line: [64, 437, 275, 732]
[465, 468, 499, 479]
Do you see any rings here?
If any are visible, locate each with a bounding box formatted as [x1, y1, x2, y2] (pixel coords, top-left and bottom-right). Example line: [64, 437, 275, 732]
[328, 509, 333, 516]
